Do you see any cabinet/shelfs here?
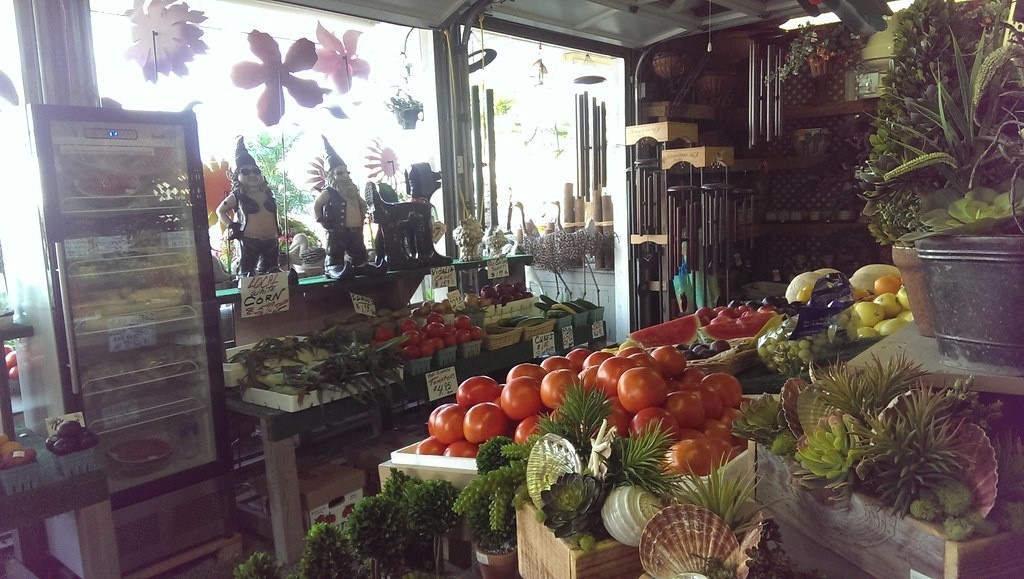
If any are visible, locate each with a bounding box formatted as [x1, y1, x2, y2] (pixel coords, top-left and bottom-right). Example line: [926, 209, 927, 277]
[0, 315, 111, 579]
[213, 254, 606, 567]
[530, 263, 616, 343]
[638, 99, 860, 238]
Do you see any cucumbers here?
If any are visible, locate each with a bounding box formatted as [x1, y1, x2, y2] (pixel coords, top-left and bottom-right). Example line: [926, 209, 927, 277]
[498, 294, 598, 328]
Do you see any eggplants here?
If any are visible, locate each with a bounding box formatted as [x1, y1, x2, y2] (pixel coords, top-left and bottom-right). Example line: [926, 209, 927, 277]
[480, 282, 534, 303]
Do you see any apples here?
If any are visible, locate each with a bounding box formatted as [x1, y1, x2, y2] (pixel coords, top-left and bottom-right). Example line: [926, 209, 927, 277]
[843, 275, 914, 339]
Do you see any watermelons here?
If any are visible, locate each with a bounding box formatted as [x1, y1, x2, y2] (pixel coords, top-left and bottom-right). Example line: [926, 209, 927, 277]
[626, 315, 702, 348]
[105, 438, 173, 476]
[697, 311, 778, 342]
[75, 172, 140, 208]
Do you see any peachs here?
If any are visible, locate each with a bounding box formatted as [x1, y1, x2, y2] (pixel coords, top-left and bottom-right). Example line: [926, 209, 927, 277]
[696, 304, 773, 325]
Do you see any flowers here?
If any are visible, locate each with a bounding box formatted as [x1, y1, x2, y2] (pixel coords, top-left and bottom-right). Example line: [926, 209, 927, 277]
[763, 21, 860, 87]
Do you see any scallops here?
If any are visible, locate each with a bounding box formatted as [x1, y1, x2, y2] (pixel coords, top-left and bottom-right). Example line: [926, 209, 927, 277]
[525, 373, 997, 579]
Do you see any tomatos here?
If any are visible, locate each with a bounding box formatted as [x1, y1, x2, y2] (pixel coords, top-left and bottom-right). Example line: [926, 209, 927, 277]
[372, 313, 482, 360]
[416, 344, 749, 477]
[0, 345, 96, 470]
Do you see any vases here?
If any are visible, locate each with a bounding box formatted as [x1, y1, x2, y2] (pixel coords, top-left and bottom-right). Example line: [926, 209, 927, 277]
[809, 57, 836, 79]
[790, 128, 831, 157]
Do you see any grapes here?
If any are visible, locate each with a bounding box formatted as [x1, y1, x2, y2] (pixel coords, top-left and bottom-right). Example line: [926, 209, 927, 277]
[757, 316, 852, 371]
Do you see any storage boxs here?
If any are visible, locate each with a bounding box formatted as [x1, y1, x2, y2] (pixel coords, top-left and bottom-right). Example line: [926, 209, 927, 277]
[515, 501, 645, 579]
[51, 448, 97, 481]
[416, 292, 540, 329]
[237, 365, 404, 413]
[221, 336, 310, 387]
[256, 429, 418, 532]
[1, 460, 41, 496]
[552, 308, 604, 332]
[406, 338, 482, 376]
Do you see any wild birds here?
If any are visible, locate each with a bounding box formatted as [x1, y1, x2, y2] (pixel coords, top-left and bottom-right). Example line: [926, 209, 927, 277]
[513, 200, 601, 309]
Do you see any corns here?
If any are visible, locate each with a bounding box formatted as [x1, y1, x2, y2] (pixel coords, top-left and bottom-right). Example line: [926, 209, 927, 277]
[254, 336, 340, 395]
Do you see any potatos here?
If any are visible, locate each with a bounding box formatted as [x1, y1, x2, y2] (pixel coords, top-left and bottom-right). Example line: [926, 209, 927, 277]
[373, 293, 491, 319]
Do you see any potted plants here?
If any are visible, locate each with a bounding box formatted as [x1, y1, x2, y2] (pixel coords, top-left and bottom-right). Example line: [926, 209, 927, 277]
[731, 351, 1024, 579]
[452, 373, 796, 579]
[387, 94, 425, 130]
[466, 436, 518, 579]
[852, 0, 1024, 376]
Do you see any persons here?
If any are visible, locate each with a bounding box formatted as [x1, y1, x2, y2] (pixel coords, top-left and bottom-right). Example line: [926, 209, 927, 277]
[314, 162, 388, 278]
[216, 164, 298, 287]
